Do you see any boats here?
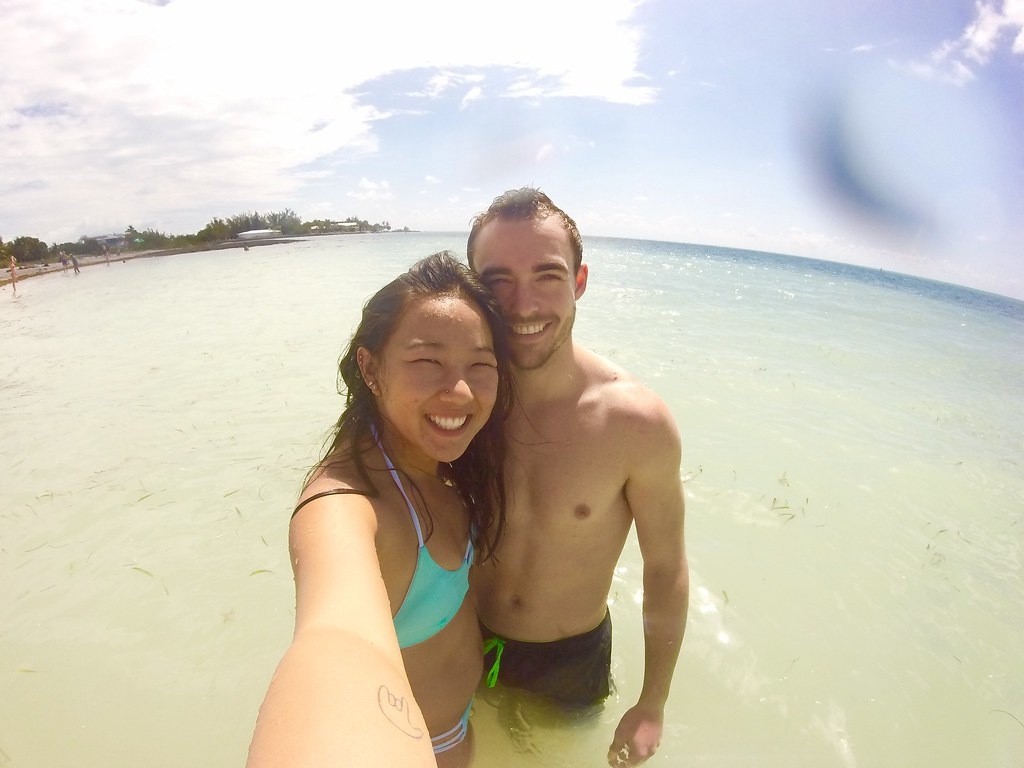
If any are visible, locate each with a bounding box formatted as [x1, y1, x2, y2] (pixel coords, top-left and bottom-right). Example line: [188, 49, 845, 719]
[235, 228, 283, 240]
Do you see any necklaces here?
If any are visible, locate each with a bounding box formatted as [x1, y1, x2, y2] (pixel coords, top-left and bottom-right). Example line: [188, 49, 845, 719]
[392, 453, 453, 487]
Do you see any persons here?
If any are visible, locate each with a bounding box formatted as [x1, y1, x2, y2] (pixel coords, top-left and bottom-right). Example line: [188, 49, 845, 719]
[9, 253, 17, 276]
[247, 249, 516, 768]
[61, 251, 68, 268]
[244, 242, 249, 251]
[468, 187, 689, 768]
[69, 254, 80, 273]
